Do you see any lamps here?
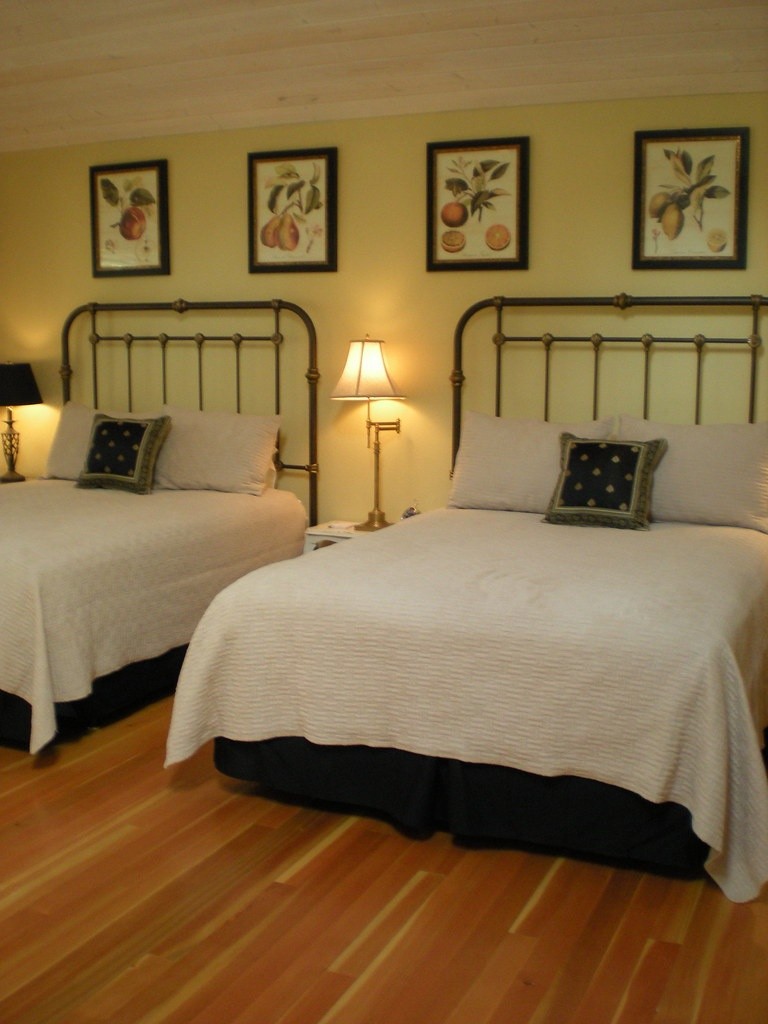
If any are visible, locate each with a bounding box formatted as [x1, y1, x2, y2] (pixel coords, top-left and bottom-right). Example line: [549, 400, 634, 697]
[330, 333, 406, 533]
[0, 361, 44, 482]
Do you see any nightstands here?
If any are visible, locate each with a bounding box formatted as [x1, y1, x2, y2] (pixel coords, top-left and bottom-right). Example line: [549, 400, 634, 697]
[1, 477, 38, 488]
[303, 519, 373, 554]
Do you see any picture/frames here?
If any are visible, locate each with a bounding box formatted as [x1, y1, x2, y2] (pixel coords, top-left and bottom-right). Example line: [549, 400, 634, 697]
[631, 125, 751, 270]
[88, 158, 171, 280]
[425, 135, 530, 272]
[248, 146, 338, 274]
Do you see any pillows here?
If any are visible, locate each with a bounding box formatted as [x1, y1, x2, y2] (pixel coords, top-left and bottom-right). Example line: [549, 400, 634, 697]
[48, 400, 158, 481]
[617, 413, 768, 534]
[542, 431, 669, 533]
[446, 409, 617, 513]
[153, 405, 282, 496]
[71, 413, 172, 495]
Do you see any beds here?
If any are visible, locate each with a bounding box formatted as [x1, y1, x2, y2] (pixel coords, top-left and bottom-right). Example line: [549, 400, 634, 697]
[214, 507, 767, 878]
[0, 477, 308, 745]
